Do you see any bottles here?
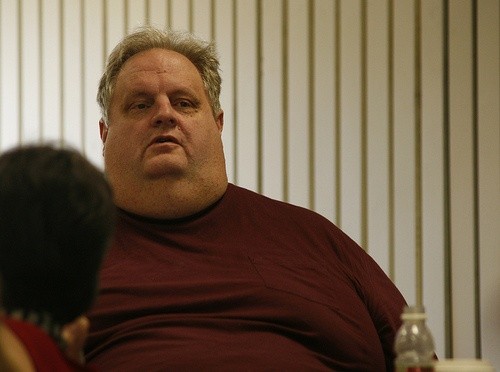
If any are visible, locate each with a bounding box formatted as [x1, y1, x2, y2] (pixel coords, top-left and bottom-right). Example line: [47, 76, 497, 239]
[392, 304, 436, 372]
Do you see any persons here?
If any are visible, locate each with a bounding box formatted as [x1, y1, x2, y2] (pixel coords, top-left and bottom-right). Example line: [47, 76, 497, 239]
[0, 139, 111, 372]
[67, 27, 439, 372]
